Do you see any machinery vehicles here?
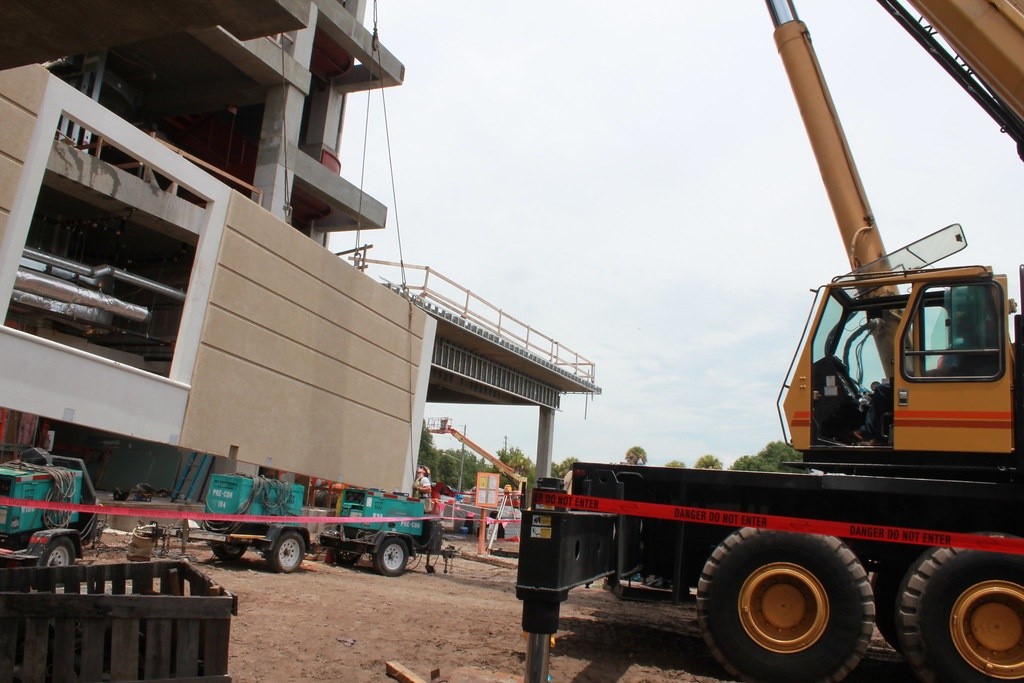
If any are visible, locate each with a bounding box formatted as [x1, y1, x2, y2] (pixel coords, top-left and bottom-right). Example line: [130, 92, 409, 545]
[320, 486, 460, 579]
[198, 472, 316, 572]
[1, 443, 103, 589]
[425, 416, 531, 511]
[509, 0, 1024, 683]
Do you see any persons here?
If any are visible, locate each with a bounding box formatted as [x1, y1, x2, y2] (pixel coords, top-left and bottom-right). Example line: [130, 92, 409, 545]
[852, 304, 973, 447]
[412, 464, 432, 514]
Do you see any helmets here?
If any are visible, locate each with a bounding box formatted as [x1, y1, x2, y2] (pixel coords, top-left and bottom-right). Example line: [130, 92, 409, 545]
[421, 466, 430, 477]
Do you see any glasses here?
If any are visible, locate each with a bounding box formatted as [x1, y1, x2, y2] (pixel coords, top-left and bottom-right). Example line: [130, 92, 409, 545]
[420, 466, 425, 471]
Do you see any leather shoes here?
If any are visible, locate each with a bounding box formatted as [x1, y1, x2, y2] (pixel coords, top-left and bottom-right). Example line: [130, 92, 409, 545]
[835, 426, 887, 445]
